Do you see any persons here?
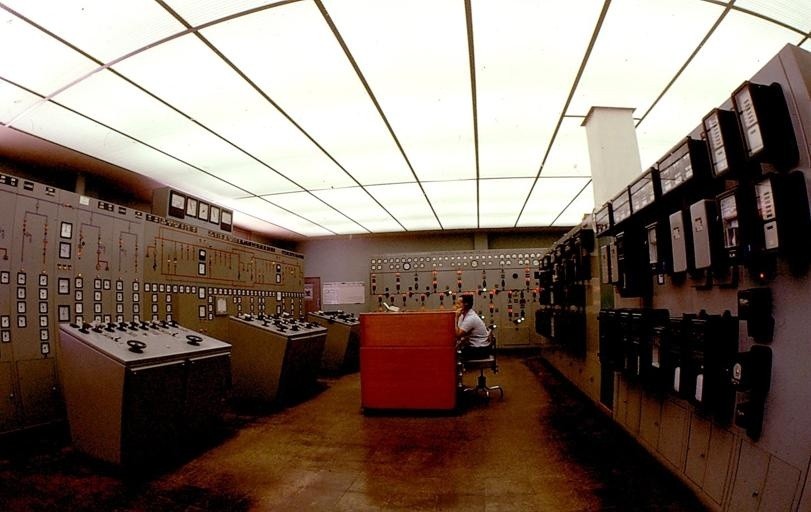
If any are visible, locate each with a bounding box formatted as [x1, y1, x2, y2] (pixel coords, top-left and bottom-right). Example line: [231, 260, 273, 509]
[455, 293, 492, 360]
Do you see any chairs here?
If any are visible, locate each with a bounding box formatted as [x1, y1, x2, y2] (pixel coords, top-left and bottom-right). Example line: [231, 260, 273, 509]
[457, 324, 504, 404]
